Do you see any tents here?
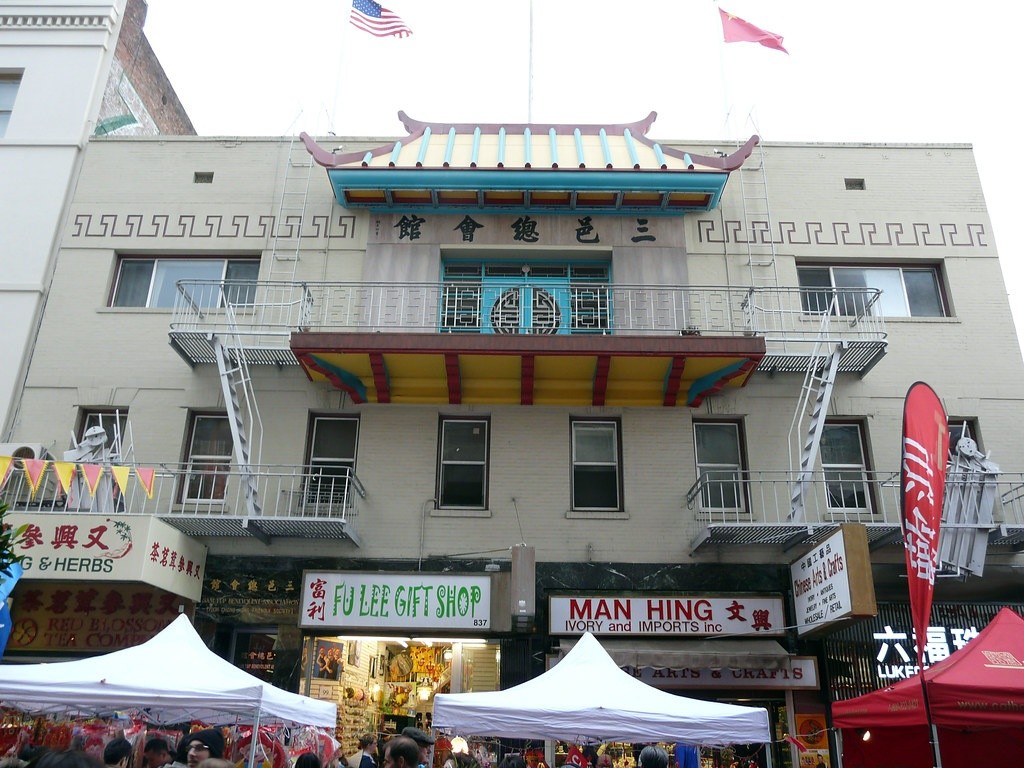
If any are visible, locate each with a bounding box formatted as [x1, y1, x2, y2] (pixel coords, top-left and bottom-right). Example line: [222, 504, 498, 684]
[832, 608, 1024, 768]
[0, 614, 337, 768]
[429, 632, 772, 768]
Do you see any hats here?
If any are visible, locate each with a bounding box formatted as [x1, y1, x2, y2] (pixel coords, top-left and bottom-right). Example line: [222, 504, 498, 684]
[347, 749, 363, 768]
[186, 729, 224, 758]
[403, 727, 434, 745]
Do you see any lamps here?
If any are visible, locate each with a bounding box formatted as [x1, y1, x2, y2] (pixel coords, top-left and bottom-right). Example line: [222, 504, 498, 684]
[427, 541, 536, 618]
[442, 648, 453, 664]
[416, 685, 433, 701]
[854, 727, 871, 742]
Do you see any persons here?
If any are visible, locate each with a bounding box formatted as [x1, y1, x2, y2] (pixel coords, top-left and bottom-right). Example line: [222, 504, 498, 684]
[817, 754, 826, 768]
[749, 755, 759, 768]
[317, 642, 342, 680]
[295, 752, 321, 768]
[498, 755, 549, 768]
[555, 740, 623, 768]
[632, 742, 669, 768]
[331, 740, 352, 768]
[104, 738, 132, 768]
[144, 729, 236, 768]
[0, 744, 103, 768]
[444, 753, 481, 768]
[348, 727, 435, 768]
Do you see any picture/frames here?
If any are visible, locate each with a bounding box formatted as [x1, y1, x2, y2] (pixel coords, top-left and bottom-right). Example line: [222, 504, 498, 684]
[309, 637, 347, 687]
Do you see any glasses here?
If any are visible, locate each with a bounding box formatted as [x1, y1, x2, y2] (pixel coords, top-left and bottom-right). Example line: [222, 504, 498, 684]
[186, 745, 209, 752]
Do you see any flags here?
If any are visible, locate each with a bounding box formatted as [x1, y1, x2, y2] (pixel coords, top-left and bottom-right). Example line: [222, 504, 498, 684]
[718, 6, 789, 55]
[350, 0, 413, 38]
